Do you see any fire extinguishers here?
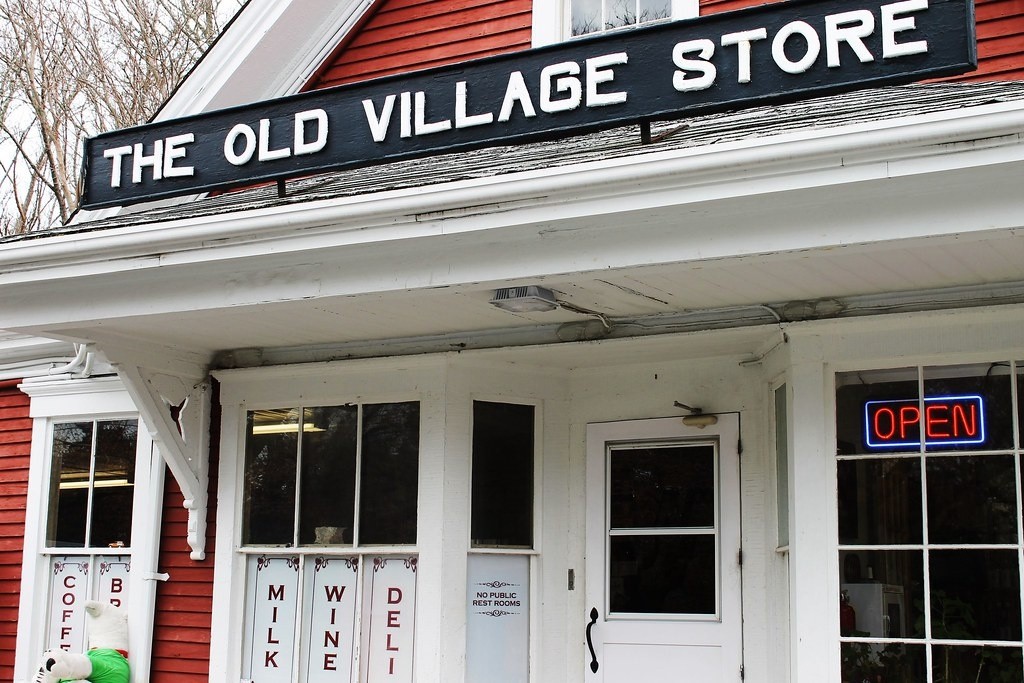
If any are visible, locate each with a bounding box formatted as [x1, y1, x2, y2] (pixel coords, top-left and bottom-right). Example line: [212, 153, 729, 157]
[840, 589, 856, 683]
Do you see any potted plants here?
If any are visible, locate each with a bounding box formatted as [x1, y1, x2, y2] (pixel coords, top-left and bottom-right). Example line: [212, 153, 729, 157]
[840, 599, 1024, 683]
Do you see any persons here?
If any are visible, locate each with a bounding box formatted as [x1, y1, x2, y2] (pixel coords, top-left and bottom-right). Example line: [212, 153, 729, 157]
[931, 508, 986, 636]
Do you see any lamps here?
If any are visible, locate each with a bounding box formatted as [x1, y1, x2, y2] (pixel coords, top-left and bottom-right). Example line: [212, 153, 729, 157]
[488, 286, 560, 312]
[253, 418, 332, 435]
[59, 475, 130, 490]
